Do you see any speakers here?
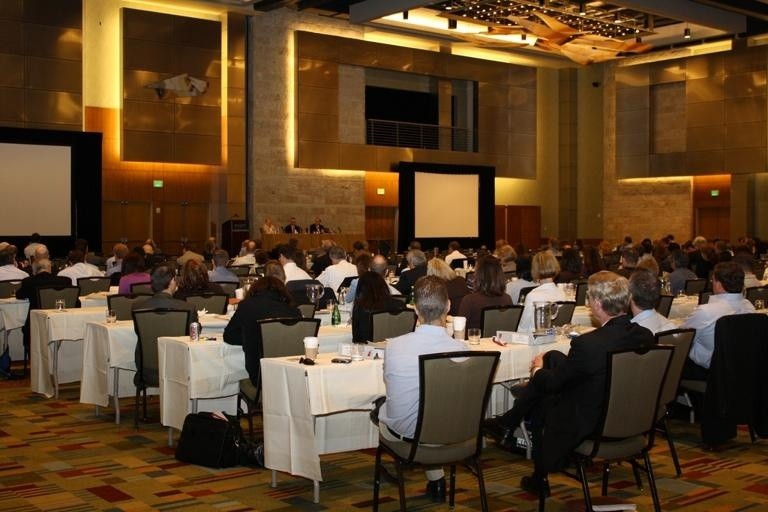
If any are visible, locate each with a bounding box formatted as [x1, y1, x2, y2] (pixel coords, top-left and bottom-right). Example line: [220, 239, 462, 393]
[222, 220, 250, 258]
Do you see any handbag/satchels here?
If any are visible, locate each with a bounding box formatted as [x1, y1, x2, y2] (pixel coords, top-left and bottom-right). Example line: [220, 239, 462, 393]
[175, 410, 245, 469]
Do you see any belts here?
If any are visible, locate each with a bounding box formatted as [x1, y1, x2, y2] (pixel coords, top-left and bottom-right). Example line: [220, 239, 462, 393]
[386, 425, 414, 443]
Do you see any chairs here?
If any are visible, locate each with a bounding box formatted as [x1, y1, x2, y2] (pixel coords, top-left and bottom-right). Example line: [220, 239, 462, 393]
[372, 351, 501, 512]
[536, 344, 675, 512]
[682, 308, 766, 446]
[604, 327, 696, 475]
[0, 229, 767, 461]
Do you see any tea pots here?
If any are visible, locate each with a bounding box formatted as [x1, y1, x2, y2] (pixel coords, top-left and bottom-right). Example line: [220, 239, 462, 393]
[304, 283, 325, 311]
[533, 301, 560, 333]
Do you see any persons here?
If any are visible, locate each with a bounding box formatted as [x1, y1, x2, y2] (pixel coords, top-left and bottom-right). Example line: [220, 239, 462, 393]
[378, 274, 478, 501]
[282, 217, 303, 235]
[108, 234, 767, 390]
[307, 216, 330, 235]
[486, 271, 655, 498]
[259, 216, 277, 235]
[1, 235, 104, 351]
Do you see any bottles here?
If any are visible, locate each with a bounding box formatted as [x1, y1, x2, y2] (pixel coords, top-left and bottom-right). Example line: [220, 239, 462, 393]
[665, 281, 671, 294]
[337, 286, 350, 313]
[226, 277, 254, 317]
[63, 262, 69, 270]
[57, 264, 62, 272]
[174, 268, 179, 278]
[331, 300, 341, 328]
[518, 293, 524, 306]
[659, 277, 666, 293]
[408, 284, 417, 305]
[618, 253, 623, 269]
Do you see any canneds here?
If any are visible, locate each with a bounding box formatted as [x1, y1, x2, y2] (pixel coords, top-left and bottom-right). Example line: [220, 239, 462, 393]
[190, 322, 200, 342]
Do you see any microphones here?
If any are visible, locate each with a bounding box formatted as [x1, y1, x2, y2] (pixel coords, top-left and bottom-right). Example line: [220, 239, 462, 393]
[281, 226, 285, 233]
[338, 227, 342, 233]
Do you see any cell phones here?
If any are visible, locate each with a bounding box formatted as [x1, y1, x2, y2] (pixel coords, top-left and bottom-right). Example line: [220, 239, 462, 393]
[331, 358, 352, 363]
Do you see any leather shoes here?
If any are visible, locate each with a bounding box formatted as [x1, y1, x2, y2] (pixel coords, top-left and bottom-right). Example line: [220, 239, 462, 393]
[520, 476, 551, 497]
[427, 480, 446, 503]
[483, 415, 517, 448]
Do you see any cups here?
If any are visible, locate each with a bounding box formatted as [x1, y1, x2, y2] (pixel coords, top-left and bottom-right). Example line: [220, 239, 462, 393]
[103, 310, 118, 325]
[466, 328, 482, 345]
[453, 315, 467, 344]
[9, 286, 17, 299]
[301, 336, 318, 361]
[754, 298, 764, 310]
[54, 299, 67, 312]
[446, 322, 454, 337]
[678, 288, 689, 298]
[338, 342, 367, 362]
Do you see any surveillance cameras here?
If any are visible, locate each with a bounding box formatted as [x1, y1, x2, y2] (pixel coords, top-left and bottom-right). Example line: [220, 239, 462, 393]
[592, 80, 601, 87]
